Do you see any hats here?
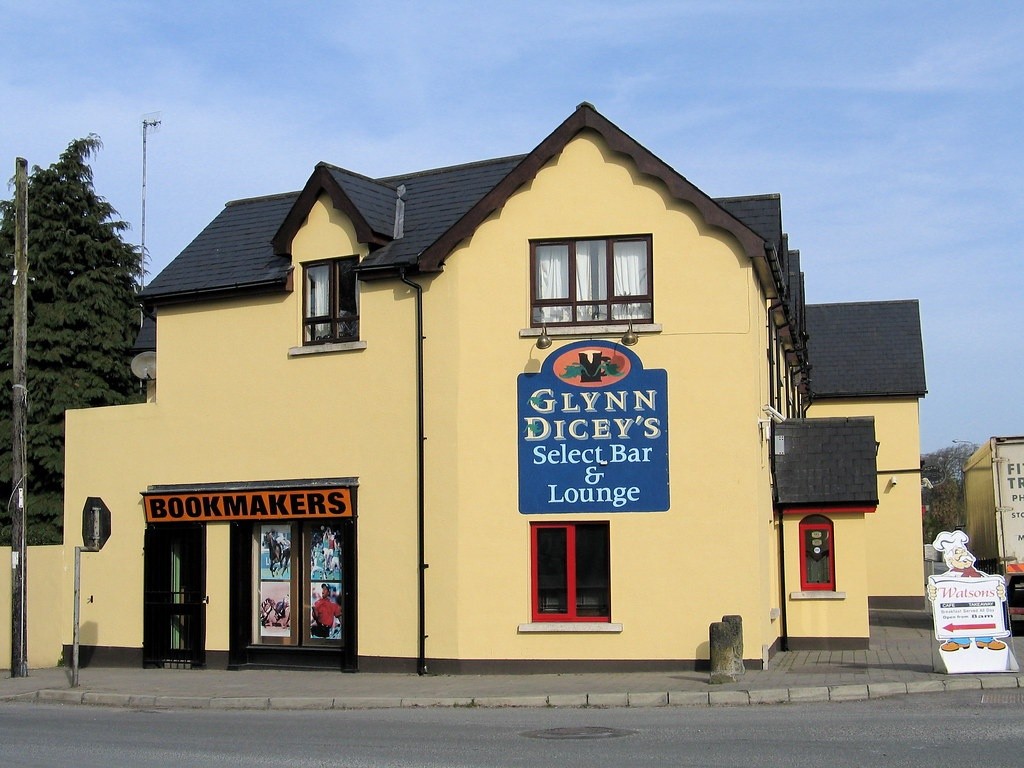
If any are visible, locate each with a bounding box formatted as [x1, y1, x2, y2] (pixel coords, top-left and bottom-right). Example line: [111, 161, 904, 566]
[321, 584, 331, 592]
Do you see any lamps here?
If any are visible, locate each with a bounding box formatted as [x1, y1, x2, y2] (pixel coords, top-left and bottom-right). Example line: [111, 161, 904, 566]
[891, 476, 896, 487]
[536, 324, 552, 349]
[621, 321, 638, 346]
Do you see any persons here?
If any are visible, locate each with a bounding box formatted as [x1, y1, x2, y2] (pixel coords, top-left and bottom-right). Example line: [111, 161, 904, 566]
[323, 528, 336, 572]
[311, 583, 342, 636]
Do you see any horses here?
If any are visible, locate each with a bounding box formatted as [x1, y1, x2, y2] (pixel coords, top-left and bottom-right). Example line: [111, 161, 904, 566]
[262, 532, 291, 577]
